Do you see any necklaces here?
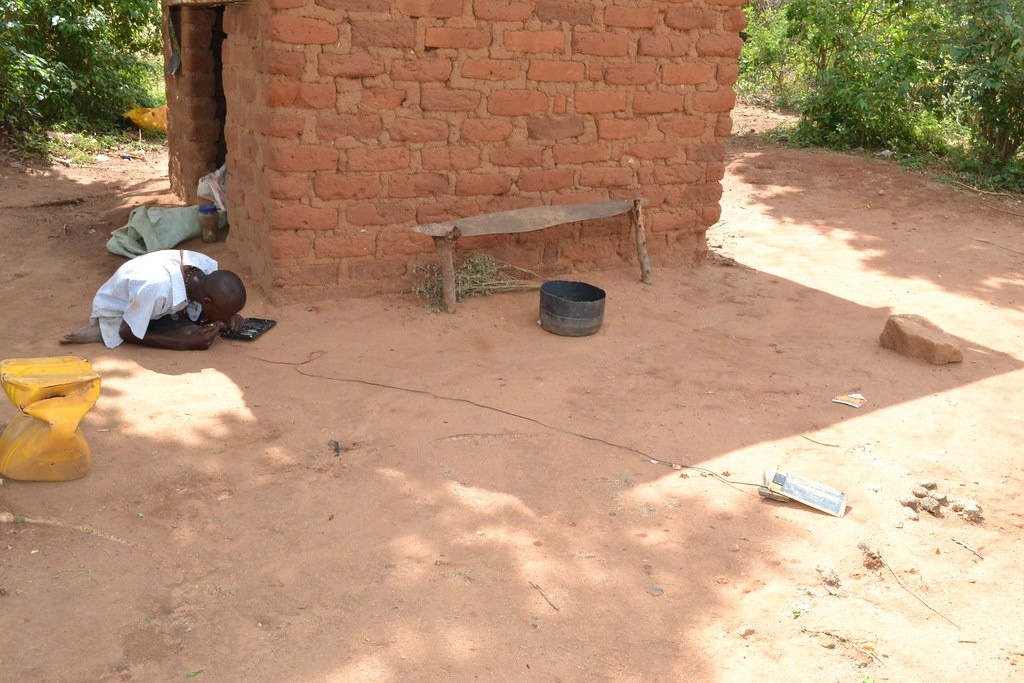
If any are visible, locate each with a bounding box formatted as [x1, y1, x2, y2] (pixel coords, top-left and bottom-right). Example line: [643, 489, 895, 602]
[185, 267, 197, 288]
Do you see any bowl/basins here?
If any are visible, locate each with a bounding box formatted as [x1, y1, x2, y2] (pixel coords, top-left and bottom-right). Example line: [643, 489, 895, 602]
[540, 281, 607, 337]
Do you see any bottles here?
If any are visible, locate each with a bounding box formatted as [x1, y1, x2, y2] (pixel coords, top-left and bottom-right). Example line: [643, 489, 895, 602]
[198, 204, 220, 244]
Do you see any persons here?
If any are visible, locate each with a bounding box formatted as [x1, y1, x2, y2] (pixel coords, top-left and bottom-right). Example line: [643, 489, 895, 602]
[59, 249, 246, 351]
